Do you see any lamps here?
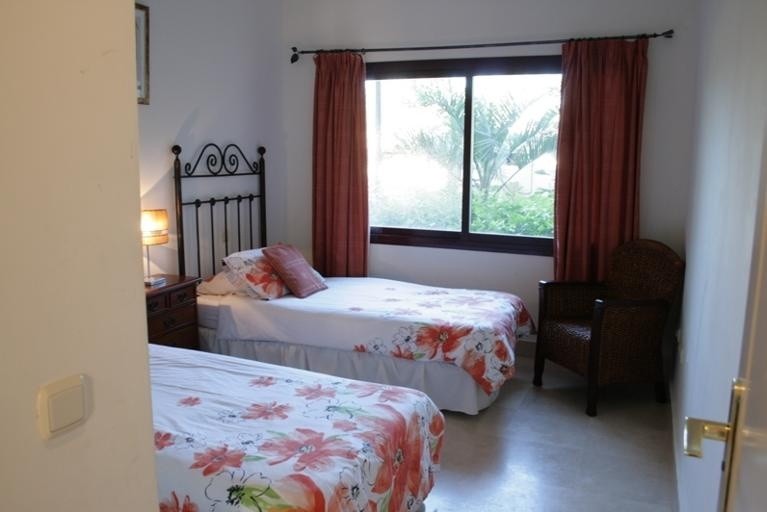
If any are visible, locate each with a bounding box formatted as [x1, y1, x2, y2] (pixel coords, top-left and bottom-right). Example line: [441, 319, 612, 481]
[140, 209, 169, 286]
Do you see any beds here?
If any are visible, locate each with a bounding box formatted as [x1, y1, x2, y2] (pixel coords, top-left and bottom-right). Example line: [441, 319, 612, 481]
[173, 142, 537, 416]
[148, 342, 447, 512]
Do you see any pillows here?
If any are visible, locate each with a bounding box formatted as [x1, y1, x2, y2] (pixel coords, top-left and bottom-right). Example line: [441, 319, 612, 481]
[261, 244, 328, 297]
[196, 270, 245, 298]
[221, 247, 326, 301]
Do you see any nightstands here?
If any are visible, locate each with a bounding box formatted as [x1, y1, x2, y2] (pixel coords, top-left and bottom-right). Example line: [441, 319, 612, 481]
[145, 273, 202, 350]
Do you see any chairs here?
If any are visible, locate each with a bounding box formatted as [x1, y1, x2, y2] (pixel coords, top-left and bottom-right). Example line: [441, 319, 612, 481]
[533, 237, 686, 417]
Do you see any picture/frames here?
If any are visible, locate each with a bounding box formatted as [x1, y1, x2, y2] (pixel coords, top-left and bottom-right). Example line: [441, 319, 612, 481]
[135, 1, 150, 103]
